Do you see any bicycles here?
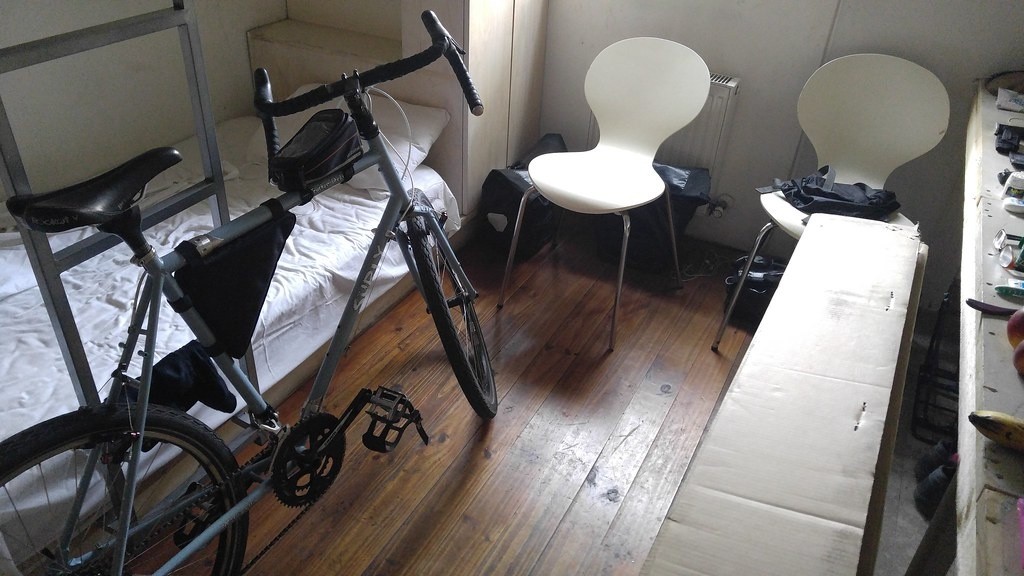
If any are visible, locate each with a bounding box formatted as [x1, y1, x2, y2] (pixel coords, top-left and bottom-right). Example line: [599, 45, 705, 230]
[0, 10, 497, 576]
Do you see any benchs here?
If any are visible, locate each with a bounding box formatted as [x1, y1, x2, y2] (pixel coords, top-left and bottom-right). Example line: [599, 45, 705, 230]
[639, 213, 929, 575]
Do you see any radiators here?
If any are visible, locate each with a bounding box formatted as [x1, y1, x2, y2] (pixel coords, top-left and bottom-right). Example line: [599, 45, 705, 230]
[586, 74, 741, 218]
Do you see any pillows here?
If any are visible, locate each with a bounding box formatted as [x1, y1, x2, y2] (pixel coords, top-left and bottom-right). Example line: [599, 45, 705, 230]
[229, 86, 454, 199]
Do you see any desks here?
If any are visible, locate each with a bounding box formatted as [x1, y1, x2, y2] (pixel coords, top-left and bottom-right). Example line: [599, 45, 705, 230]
[902, 74, 1023, 576]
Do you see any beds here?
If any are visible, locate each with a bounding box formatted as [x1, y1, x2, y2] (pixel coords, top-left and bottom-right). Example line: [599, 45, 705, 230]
[0, 112, 441, 565]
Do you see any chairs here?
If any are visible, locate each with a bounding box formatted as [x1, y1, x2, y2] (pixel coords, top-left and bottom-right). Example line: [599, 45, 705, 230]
[497, 38, 709, 350]
[713, 52, 951, 351]
